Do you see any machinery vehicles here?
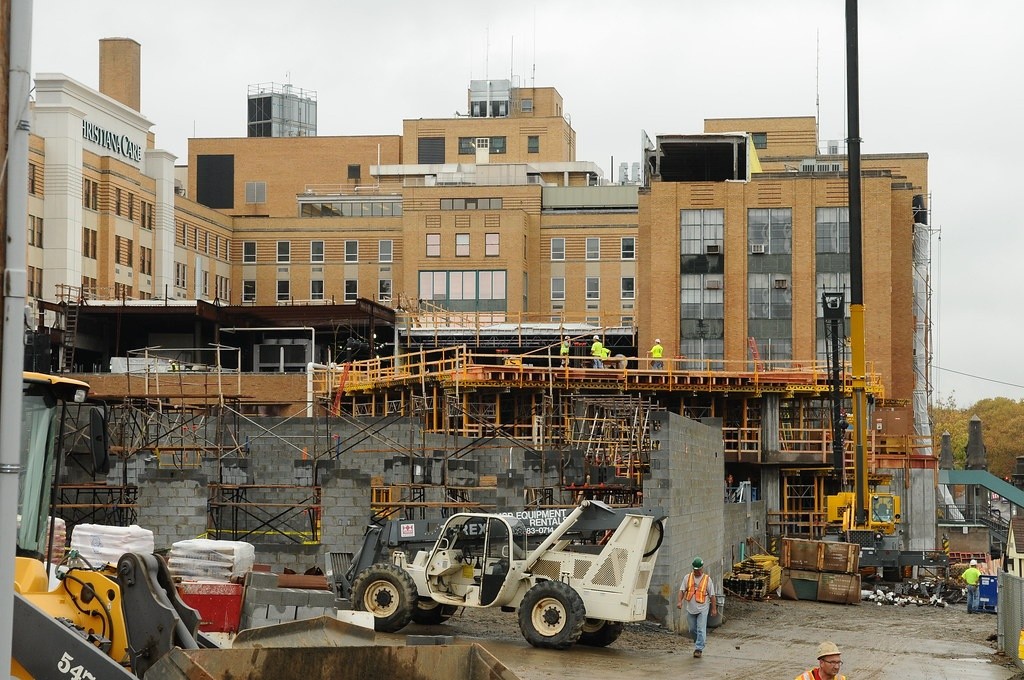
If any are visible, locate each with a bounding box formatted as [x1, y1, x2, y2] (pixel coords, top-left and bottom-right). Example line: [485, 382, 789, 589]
[344, 499, 668, 651]
[11, 371, 516, 680]
[820, 0, 949, 581]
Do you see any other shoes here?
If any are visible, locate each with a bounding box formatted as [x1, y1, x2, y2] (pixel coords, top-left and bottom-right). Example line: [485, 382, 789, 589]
[694, 651, 701, 657]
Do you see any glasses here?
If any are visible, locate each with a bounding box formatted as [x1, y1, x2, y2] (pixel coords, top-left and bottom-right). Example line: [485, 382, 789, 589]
[823, 660, 843, 666]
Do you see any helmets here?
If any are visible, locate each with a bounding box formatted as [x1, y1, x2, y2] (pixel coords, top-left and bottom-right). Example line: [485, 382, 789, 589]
[655, 339, 660, 342]
[565, 336, 570, 340]
[593, 336, 599, 340]
[970, 560, 977, 565]
[692, 557, 703, 570]
[817, 642, 841, 659]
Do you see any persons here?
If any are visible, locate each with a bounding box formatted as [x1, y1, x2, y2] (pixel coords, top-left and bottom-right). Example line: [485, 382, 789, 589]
[794, 641, 847, 680]
[646, 339, 663, 371]
[960, 559, 982, 614]
[677, 557, 718, 658]
[590, 335, 611, 369]
[560, 336, 571, 369]
